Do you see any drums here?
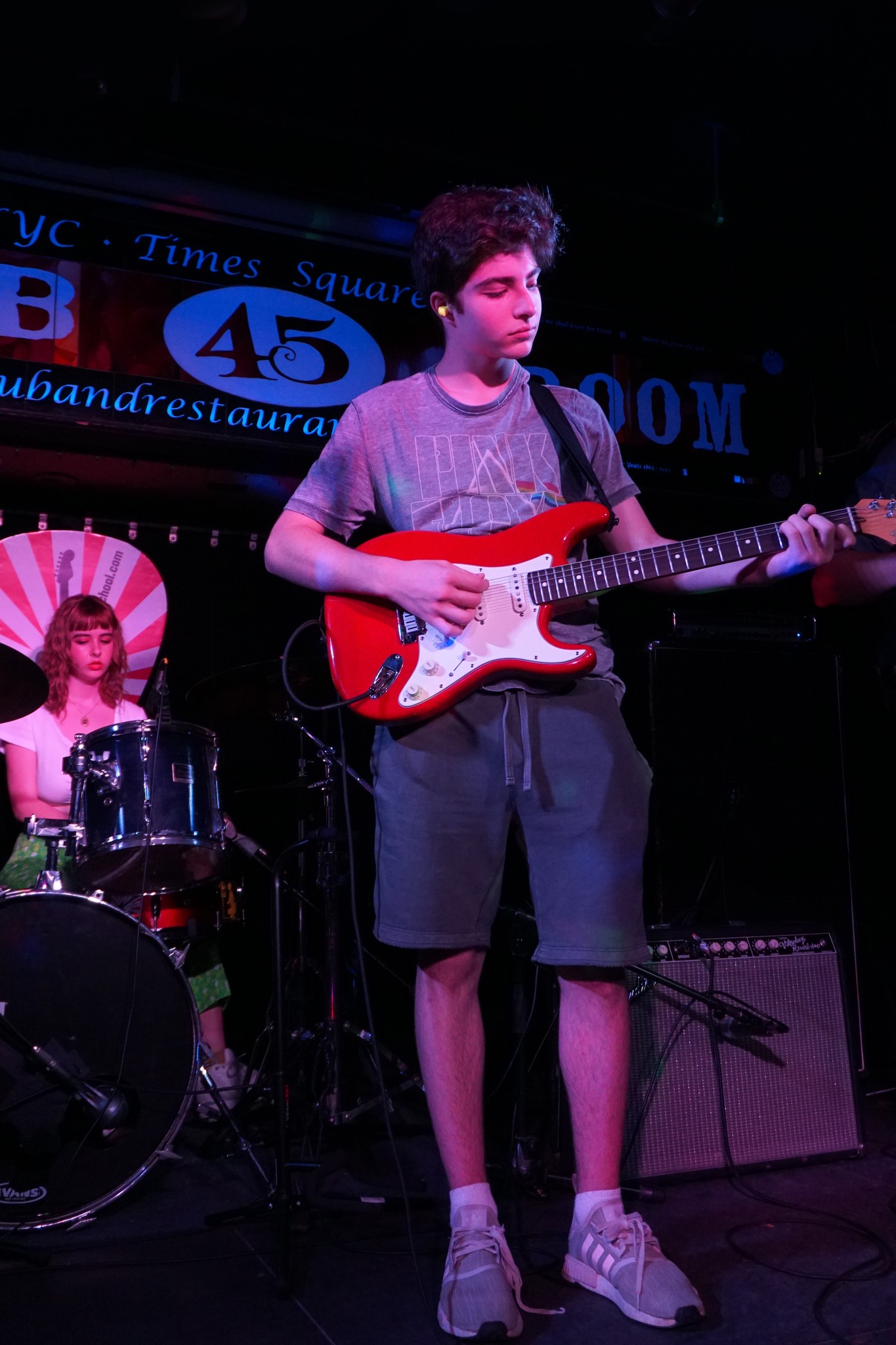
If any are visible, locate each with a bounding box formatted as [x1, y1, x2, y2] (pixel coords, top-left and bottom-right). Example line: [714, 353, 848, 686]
[0, 886, 203, 1231]
[61, 715, 225, 888]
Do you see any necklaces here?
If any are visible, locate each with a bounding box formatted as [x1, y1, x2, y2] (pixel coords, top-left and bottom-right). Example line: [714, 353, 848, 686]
[67, 696, 102, 727]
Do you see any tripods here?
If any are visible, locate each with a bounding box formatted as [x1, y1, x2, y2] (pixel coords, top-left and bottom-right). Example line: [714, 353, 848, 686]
[201, 709, 426, 1298]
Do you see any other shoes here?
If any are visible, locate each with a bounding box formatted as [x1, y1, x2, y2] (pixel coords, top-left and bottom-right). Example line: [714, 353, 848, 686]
[201, 1050, 246, 1121]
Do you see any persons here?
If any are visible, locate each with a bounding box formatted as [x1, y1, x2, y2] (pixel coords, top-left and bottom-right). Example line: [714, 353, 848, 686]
[0, 594, 259, 1119]
[810, 449, 896, 608]
[263, 181, 855, 1345]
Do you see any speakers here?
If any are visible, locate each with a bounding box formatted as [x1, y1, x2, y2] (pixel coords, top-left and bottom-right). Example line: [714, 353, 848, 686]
[622, 932, 866, 1189]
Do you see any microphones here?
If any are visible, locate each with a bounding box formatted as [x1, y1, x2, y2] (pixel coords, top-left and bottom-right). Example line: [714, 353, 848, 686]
[35, 1047, 129, 1127]
[147, 658, 166, 719]
[221, 817, 271, 863]
[720, 1017, 789, 1039]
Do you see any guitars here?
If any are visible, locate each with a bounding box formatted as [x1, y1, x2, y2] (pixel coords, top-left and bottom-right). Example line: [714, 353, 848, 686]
[323, 494, 896, 722]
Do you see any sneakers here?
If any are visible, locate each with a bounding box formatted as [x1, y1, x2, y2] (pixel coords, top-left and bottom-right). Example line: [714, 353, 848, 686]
[561, 1195, 705, 1330]
[438, 1207, 568, 1340]
